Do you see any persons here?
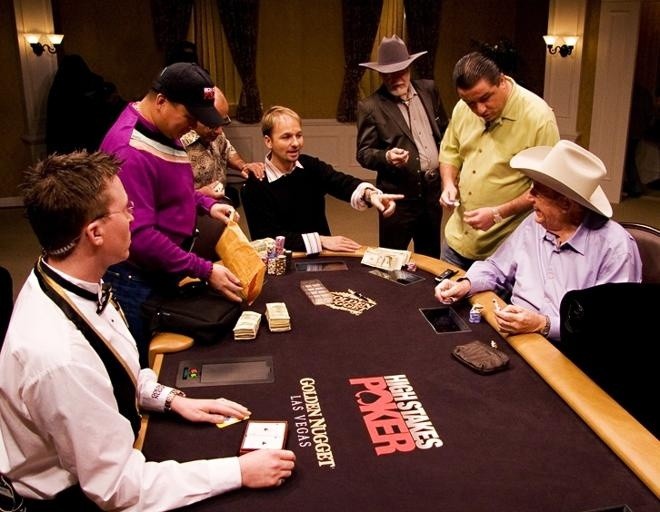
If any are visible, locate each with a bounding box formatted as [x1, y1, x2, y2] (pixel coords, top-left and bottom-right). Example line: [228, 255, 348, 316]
[240, 106, 405, 256]
[356, 34, 449, 259]
[0, 149, 297, 512]
[45, 54, 128, 156]
[438, 51, 560, 303]
[434, 139, 643, 339]
[98, 62, 241, 369]
[177, 85, 265, 200]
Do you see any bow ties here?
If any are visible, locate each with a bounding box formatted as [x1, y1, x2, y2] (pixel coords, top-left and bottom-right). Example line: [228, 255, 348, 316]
[95, 276, 118, 314]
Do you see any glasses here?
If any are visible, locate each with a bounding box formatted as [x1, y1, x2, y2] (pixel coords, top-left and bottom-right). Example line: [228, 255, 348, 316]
[89, 200, 135, 224]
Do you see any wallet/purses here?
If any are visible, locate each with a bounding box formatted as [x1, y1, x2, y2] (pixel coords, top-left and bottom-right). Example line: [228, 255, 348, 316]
[451, 340, 510, 375]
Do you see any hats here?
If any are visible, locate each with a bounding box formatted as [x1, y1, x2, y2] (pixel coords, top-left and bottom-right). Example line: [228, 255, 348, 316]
[150, 62, 224, 128]
[358, 34, 428, 73]
[510, 139, 613, 217]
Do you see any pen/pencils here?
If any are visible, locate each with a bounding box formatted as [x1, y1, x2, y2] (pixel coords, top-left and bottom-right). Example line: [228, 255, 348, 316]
[348, 289, 369, 301]
[492, 300, 500, 312]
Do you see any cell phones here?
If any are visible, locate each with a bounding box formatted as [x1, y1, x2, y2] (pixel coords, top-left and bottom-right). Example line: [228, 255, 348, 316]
[434, 268, 459, 283]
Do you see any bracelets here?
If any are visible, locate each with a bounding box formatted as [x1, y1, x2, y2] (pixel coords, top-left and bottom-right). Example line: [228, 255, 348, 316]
[541, 316, 550, 337]
[165, 389, 186, 414]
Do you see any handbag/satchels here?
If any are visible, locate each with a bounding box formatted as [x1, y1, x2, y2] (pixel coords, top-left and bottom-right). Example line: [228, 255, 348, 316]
[215, 220, 267, 306]
[139, 283, 241, 345]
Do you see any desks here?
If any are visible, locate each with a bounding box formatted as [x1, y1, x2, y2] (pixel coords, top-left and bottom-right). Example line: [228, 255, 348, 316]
[133, 245, 660, 512]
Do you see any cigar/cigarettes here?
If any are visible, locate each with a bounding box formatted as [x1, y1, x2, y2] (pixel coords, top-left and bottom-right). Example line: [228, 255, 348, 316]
[493, 299, 499, 310]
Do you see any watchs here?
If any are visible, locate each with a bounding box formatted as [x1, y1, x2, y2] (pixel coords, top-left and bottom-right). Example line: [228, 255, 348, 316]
[492, 206, 503, 225]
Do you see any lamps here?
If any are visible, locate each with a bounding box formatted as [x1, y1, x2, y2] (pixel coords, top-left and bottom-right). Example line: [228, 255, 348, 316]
[542, 34, 579, 57]
[23, 33, 64, 57]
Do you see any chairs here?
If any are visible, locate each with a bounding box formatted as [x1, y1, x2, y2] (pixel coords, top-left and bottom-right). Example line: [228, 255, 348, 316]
[613, 222, 660, 286]
